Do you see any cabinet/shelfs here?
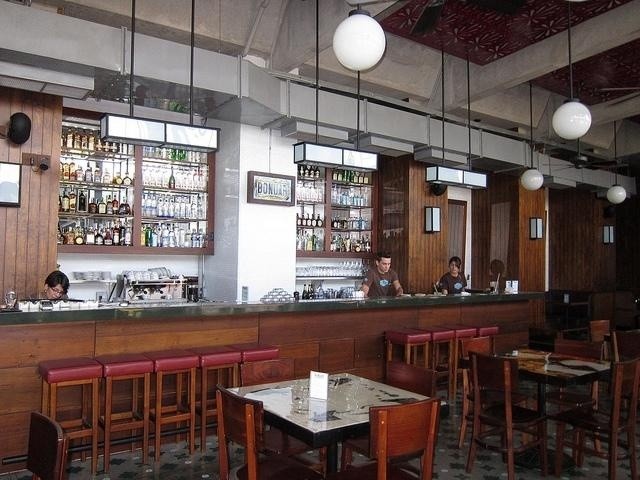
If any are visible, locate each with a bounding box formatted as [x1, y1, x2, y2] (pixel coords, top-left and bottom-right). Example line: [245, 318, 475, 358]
[58, 113, 213, 255]
[296, 145, 379, 257]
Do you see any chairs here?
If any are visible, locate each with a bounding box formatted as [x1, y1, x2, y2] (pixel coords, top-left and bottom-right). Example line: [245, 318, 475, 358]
[24, 410, 70, 480]
[341, 362, 440, 473]
[338, 396, 442, 479]
[240, 358, 327, 477]
[457, 319, 640, 480]
[215, 382, 326, 479]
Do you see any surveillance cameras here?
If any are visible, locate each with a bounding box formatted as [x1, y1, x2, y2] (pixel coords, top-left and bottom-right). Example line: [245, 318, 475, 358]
[38, 158, 50, 171]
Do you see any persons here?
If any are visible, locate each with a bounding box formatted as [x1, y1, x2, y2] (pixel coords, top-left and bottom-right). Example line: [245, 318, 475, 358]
[360, 252, 403, 297]
[438, 256, 467, 294]
[29, 270, 70, 299]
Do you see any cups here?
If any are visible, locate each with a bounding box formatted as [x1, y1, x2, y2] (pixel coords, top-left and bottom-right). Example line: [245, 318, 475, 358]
[73, 270, 111, 282]
[490, 282, 498, 295]
[260, 288, 295, 303]
[124, 271, 159, 280]
[297, 261, 368, 277]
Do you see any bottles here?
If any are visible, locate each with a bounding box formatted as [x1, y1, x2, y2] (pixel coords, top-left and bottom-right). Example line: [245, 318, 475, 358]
[301, 283, 314, 301]
[298, 165, 373, 253]
[139, 144, 210, 248]
[59, 128, 135, 246]
[315, 287, 355, 299]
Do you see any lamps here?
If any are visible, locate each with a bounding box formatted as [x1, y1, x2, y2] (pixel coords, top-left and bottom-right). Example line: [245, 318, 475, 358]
[291, 0, 379, 173]
[30, 156, 50, 174]
[603, 223, 615, 245]
[521, 82, 543, 193]
[329, 1, 387, 75]
[424, 205, 442, 234]
[551, 0, 594, 141]
[425, 42, 488, 192]
[529, 216, 544, 241]
[606, 120, 629, 206]
[98, 0, 222, 155]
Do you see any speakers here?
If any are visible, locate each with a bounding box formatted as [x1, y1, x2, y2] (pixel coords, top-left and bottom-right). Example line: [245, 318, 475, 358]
[9, 111, 31, 144]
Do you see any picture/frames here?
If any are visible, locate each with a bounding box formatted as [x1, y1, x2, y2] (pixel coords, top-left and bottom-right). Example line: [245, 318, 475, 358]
[0, 161, 22, 209]
[247, 171, 296, 207]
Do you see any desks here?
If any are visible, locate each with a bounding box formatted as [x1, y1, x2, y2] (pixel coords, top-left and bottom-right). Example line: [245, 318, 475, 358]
[226, 373, 446, 479]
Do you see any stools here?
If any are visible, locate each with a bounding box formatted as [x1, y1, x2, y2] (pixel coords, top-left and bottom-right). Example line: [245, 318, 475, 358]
[385, 328, 432, 369]
[233, 343, 281, 387]
[427, 326, 457, 398]
[37, 344, 241, 475]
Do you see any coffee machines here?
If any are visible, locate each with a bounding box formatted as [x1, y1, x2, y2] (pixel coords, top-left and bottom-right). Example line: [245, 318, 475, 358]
[182, 275, 204, 304]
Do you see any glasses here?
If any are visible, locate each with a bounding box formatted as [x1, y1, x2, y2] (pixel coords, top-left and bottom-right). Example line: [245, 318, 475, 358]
[49, 286, 66, 296]
